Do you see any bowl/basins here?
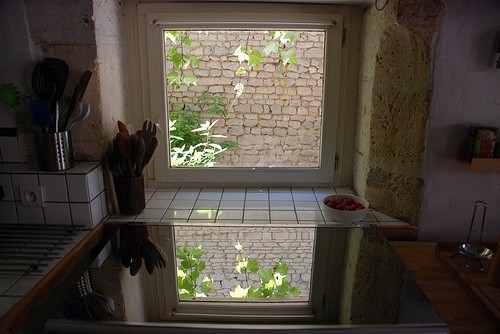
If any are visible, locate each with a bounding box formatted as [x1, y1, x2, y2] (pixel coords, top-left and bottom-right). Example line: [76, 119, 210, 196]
[322, 193, 370, 222]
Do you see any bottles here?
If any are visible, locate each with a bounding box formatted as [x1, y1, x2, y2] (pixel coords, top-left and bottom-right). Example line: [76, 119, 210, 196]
[486, 245, 500, 289]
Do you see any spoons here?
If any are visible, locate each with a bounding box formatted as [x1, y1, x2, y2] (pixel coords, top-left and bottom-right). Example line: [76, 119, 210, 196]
[64, 102, 82, 131]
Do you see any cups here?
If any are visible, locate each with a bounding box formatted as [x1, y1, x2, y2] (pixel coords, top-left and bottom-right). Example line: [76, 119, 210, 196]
[114, 173, 145, 215]
[120, 223, 148, 246]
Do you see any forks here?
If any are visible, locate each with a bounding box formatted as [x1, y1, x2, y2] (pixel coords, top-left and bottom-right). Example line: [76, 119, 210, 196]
[67, 102, 90, 131]
